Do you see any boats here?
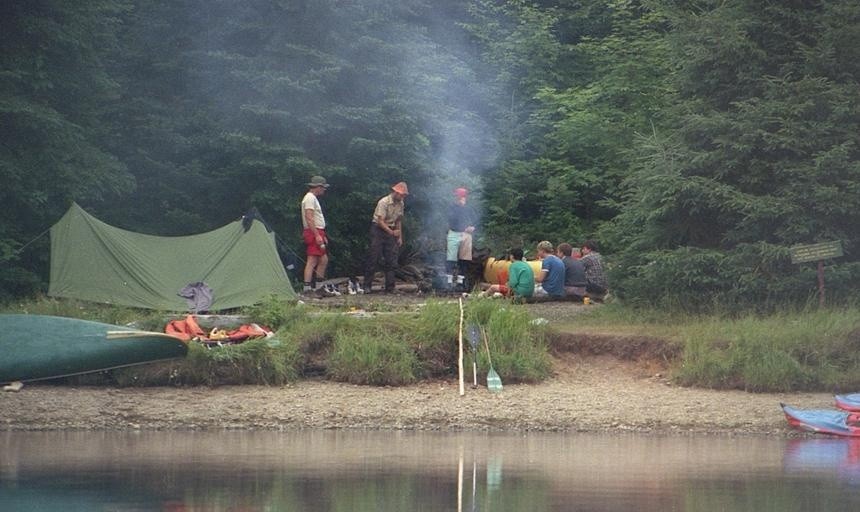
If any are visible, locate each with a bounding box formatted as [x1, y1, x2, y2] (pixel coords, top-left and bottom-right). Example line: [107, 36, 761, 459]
[778, 401, 860, 437]
[484, 256, 542, 285]
[833, 392, 859, 411]
[1, 312, 190, 386]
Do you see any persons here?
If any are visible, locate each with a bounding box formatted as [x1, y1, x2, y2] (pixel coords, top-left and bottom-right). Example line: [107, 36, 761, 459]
[298, 174, 338, 298]
[363, 180, 410, 293]
[461, 247, 535, 305]
[576, 237, 609, 293]
[532, 239, 566, 296]
[445, 186, 478, 288]
[557, 242, 586, 298]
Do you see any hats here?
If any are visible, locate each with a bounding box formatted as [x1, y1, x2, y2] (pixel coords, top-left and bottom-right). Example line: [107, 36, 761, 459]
[452, 188, 467, 197]
[391, 181, 410, 195]
[304, 175, 331, 188]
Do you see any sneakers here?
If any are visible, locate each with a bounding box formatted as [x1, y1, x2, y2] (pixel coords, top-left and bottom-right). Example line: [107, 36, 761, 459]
[447, 283, 465, 292]
[303, 286, 336, 299]
[385, 288, 401, 296]
[362, 284, 372, 294]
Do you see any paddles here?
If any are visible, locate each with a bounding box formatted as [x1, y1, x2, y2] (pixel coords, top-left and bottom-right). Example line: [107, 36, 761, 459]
[482, 326, 504, 390]
[468, 325, 480, 387]
